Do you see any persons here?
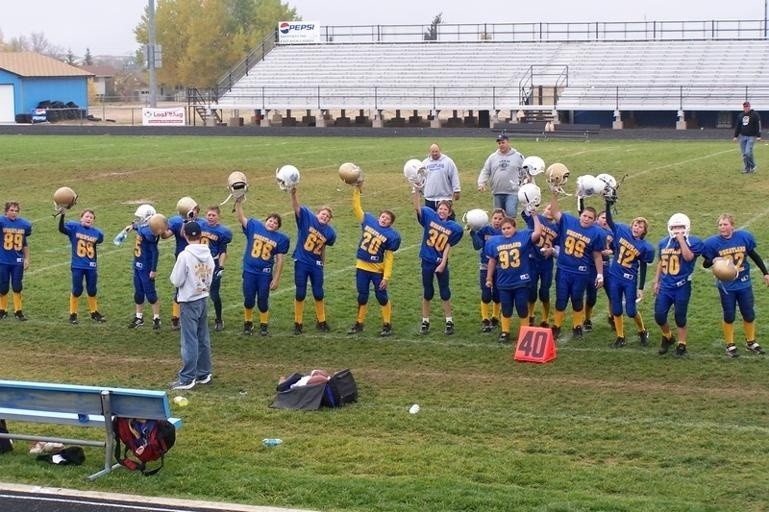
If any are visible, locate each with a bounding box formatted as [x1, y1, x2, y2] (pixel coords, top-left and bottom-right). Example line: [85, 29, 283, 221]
[59, 207, 106, 324]
[412, 184, 463, 335]
[347, 180, 401, 337]
[470, 180, 769, 359]
[167, 221, 216, 391]
[733, 102, 762, 173]
[477, 134, 535, 220]
[419, 144, 461, 221]
[235, 195, 289, 337]
[290, 187, 336, 335]
[0, 201, 31, 321]
[127, 199, 232, 333]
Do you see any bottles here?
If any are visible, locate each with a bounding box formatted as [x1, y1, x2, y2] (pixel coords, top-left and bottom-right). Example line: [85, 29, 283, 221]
[174, 396, 188, 407]
[113, 231, 128, 247]
[262, 439, 283, 445]
[409, 404, 420, 414]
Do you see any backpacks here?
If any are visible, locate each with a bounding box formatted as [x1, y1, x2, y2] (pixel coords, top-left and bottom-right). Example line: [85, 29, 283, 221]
[112, 417, 176, 477]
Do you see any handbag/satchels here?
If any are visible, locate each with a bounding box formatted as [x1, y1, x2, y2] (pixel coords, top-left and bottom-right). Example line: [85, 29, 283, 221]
[267, 367, 357, 411]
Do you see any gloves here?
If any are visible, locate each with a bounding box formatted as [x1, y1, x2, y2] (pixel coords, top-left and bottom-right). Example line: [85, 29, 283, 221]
[214, 266, 224, 282]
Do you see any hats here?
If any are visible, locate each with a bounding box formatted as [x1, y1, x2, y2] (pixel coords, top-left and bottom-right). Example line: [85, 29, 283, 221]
[183, 222, 201, 236]
[495, 135, 509, 142]
[743, 102, 750, 105]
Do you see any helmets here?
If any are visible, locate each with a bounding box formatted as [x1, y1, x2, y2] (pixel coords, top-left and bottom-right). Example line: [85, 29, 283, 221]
[176, 197, 198, 220]
[53, 187, 78, 210]
[467, 156, 618, 233]
[133, 204, 156, 228]
[667, 213, 690, 238]
[338, 163, 361, 184]
[150, 215, 169, 235]
[276, 165, 300, 190]
[404, 159, 427, 184]
[227, 171, 248, 194]
[711, 259, 740, 281]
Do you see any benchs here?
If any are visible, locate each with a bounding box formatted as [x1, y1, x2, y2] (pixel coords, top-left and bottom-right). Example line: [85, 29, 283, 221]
[0, 379, 181, 483]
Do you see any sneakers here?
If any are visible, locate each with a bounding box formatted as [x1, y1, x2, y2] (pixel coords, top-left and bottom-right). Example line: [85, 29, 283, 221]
[529, 315, 767, 358]
[152, 318, 161, 330]
[90, 310, 106, 323]
[741, 170, 750, 174]
[68, 312, 79, 324]
[128, 317, 144, 329]
[196, 373, 213, 384]
[0, 309, 9, 320]
[169, 379, 196, 390]
[14, 310, 28, 322]
[750, 167, 756, 175]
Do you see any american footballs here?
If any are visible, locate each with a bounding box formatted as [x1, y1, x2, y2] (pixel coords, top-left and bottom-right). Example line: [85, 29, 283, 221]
[310, 368, 327, 375]
[307, 374, 328, 384]
